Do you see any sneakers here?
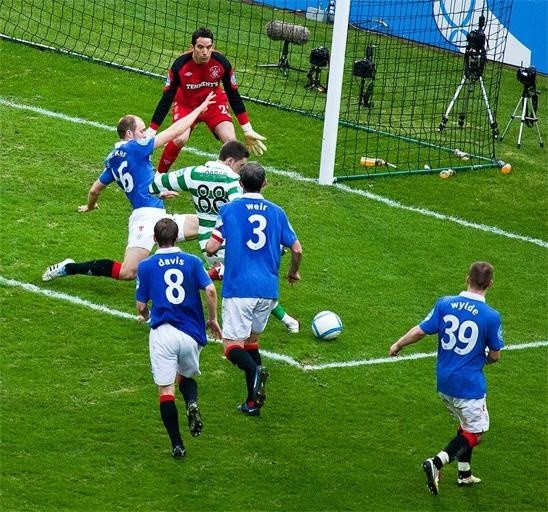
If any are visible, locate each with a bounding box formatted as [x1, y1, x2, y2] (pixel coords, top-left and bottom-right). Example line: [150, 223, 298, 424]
[41, 257, 75, 282]
[290, 320, 300, 334]
[170, 446, 187, 460]
[456, 473, 483, 486]
[251, 367, 268, 407]
[422, 457, 442, 495]
[185, 407, 205, 438]
[241, 403, 260, 415]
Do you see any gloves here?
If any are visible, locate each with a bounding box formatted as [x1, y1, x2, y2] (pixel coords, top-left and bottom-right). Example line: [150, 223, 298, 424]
[241, 122, 267, 158]
[144, 129, 156, 141]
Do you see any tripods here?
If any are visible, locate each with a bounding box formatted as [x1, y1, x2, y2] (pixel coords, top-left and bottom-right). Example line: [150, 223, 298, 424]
[255, 40, 308, 77]
[437, 67, 499, 140]
[500, 94, 544, 150]
[303, 67, 327, 94]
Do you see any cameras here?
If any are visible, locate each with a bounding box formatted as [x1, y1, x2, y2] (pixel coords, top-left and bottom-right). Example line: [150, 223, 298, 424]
[309, 45, 330, 67]
[516, 60, 536, 87]
[352, 45, 376, 79]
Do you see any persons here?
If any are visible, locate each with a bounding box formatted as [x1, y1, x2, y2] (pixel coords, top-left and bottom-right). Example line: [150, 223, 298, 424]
[145, 26, 267, 178]
[41, 91, 217, 283]
[204, 160, 302, 416]
[387, 261, 503, 496]
[147, 140, 301, 333]
[134, 217, 223, 458]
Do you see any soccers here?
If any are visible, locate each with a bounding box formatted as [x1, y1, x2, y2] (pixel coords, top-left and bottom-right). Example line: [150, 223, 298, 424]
[309, 310, 343, 341]
